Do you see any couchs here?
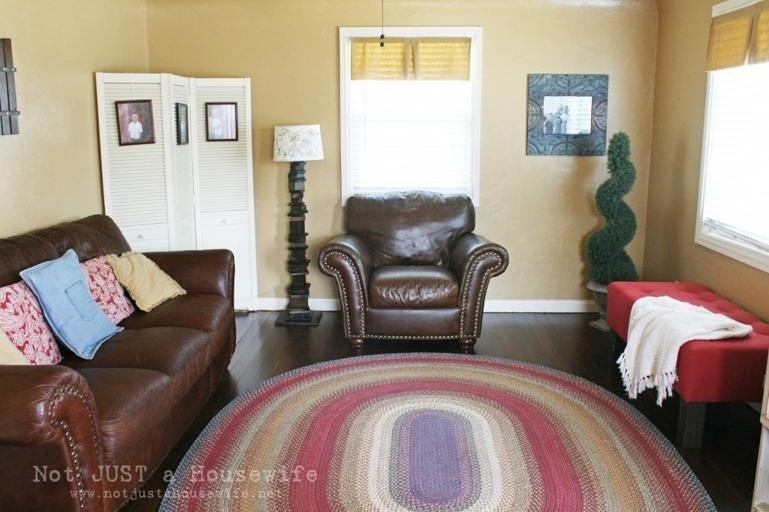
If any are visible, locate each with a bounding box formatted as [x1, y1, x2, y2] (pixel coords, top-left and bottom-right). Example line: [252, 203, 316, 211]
[1, 212, 237, 512]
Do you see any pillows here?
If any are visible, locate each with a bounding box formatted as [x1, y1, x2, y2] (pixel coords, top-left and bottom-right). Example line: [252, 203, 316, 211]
[0, 279, 64, 367]
[19, 247, 125, 361]
[102, 251, 187, 313]
[80, 254, 135, 326]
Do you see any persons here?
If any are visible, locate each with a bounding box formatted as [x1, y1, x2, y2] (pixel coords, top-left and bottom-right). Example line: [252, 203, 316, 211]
[544, 104, 570, 134]
[127, 112, 144, 142]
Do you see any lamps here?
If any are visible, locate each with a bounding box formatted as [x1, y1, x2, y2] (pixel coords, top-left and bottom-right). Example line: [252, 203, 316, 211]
[271, 122, 324, 326]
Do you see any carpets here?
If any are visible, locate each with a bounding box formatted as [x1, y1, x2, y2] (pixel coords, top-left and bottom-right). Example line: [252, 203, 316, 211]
[158, 348, 717, 511]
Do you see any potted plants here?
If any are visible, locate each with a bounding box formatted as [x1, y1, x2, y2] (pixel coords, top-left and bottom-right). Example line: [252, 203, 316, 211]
[584, 128, 639, 331]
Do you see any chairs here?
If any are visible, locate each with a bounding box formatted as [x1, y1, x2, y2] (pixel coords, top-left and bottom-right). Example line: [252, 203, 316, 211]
[317, 190, 509, 356]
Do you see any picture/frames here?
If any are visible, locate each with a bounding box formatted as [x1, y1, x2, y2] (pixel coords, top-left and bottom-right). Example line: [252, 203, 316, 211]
[527, 73, 609, 157]
[115, 99, 156, 146]
[205, 102, 238, 142]
[177, 102, 189, 144]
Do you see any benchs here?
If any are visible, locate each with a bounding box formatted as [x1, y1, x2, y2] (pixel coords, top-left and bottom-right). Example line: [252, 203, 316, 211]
[604, 279, 769, 472]
